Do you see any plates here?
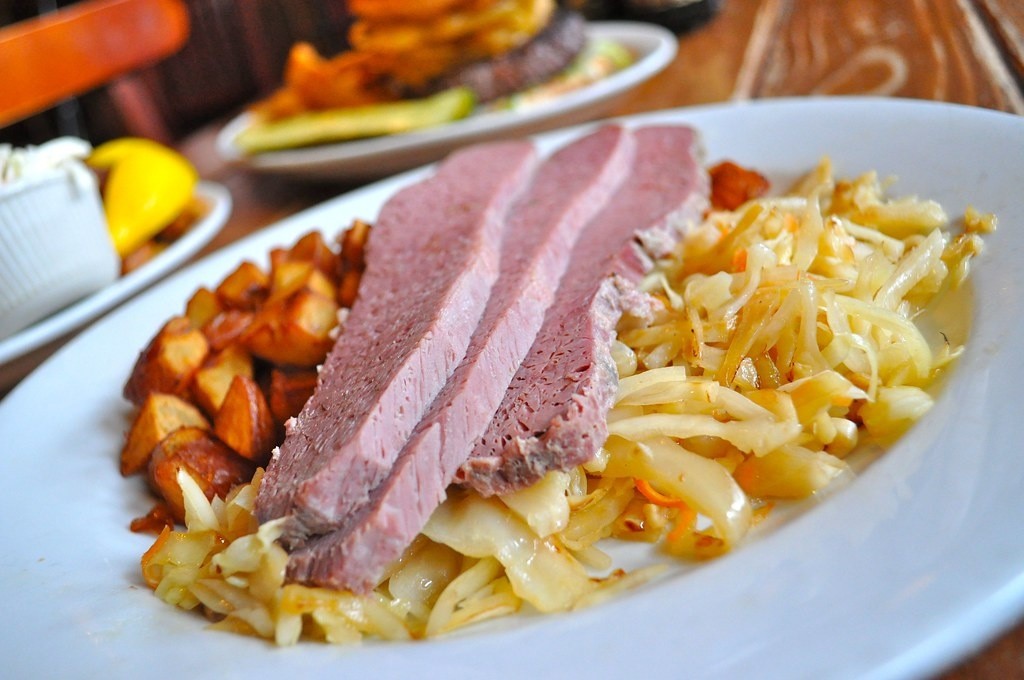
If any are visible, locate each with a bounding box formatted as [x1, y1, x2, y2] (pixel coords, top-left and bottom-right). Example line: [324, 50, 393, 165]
[0, 181, 231, 395]
[214, 16, 678, 191]
[0, 97, 1023, 679]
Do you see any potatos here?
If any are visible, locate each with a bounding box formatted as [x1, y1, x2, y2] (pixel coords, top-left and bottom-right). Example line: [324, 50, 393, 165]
[119, 220, 370, 524]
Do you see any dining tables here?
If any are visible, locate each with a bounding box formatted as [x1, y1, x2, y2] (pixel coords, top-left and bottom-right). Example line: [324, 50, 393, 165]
[0, 1, 1024, 680]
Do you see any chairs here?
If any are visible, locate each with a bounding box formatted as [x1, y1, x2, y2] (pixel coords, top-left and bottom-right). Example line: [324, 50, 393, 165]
[0, 0, 191, 147]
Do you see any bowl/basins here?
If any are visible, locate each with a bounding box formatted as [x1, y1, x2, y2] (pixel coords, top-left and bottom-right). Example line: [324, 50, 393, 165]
[1, 173, 120, 339]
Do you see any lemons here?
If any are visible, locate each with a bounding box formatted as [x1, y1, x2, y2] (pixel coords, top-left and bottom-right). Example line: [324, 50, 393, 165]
[85, 137, 199, 252]
